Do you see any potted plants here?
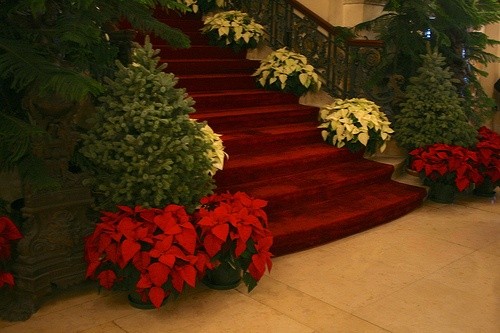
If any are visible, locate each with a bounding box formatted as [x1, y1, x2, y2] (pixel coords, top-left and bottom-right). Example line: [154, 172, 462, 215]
[393, 48, 478, 175]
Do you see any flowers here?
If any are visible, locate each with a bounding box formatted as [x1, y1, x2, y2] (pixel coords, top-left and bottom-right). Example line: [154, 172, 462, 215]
[250, 47, 323, 97]
[194, 191, 274, 292]
[408, 126, 500, 192]
[198, 11, 268, 52]
[182, 0, 230, 14]
[317, 97, 394, 153]
[0, 216, 22, 293]
[189, 119, 229, 177]
[80, 204, 205, 310]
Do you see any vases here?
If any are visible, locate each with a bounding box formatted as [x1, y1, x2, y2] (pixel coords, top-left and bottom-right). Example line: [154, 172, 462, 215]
[129, 281, 171, 305]
[348, 141, 373, 157]
[207, 260, 241, 286]
[475, 180, 497, 197]
[427, 182, 457, 204]
